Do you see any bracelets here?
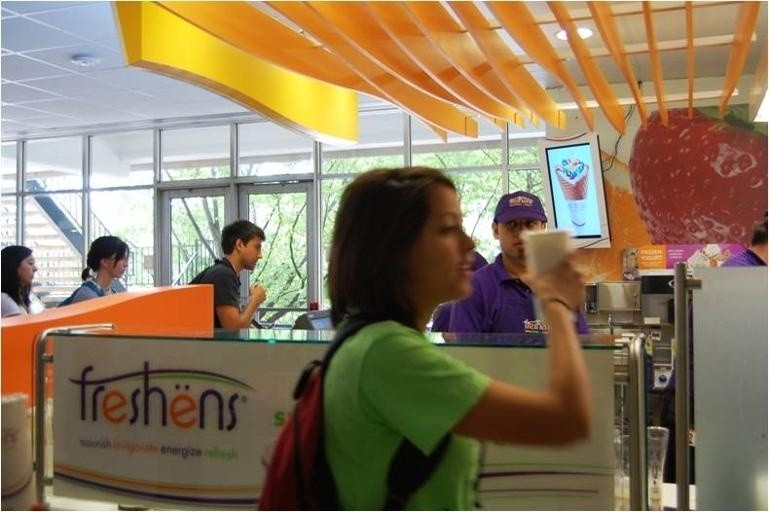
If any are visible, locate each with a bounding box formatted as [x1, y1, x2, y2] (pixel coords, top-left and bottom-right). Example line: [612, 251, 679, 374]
[539, 297, 572, 312]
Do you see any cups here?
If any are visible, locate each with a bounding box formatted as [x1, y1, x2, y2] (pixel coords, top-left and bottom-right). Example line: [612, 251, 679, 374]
[520, 231, 572, 329]
[309, 300, 319, 311]
[646, 426, 671, 511]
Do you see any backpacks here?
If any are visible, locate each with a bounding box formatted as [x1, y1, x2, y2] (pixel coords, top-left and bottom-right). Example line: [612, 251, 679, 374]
[257, 311, 451, 511]
[56, 283, 118, 307]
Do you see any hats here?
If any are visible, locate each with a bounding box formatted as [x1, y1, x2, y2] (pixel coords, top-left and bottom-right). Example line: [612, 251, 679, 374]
[495, 190, 547, 224]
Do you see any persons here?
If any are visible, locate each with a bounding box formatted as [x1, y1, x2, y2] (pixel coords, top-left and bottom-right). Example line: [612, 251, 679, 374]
[70, 236, 129, 304]
[432, 250, 488, 332]
[660, 209, 768, 483]
[322, 166, 595, 511]
[1, 246, 45, 318]
[190, 221, 266, 329]
[448, 189, 590, 336]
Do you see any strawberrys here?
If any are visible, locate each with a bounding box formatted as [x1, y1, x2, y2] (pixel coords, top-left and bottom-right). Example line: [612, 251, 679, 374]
[629, 109, 768, 245]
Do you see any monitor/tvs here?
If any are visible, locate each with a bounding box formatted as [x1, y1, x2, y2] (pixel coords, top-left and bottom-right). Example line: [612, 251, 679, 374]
[539, 134, 611, 249]
[291, 308, 335, 331]
